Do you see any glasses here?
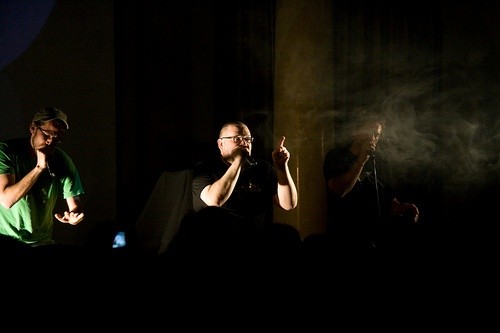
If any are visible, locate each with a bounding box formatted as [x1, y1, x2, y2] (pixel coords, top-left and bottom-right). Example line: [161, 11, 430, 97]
[219, 136, 254, 144]
[39, 127, 62, 144]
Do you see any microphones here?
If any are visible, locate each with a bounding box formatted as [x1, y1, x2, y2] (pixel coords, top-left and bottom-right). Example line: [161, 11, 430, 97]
[45, 159, 56, 177]
[242, 151, 258, 165]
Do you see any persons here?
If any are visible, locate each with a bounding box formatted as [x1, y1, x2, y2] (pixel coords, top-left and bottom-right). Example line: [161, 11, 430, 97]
[0, 106, 85, 247]
[192, 121, 298, 226]
[324, 113, 419, 241]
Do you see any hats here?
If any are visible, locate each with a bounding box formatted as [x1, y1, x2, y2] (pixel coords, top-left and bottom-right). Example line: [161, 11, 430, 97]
[33, 106, 68, 129]
